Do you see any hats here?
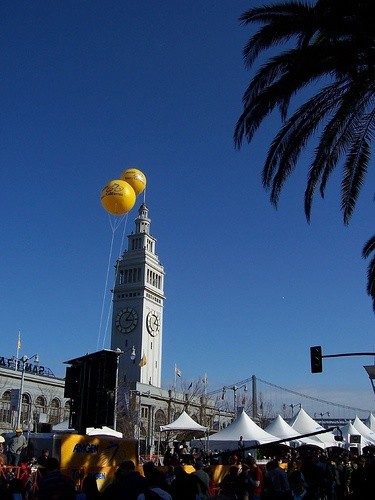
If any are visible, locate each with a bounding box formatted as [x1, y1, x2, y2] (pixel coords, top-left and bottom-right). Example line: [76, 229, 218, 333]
[40, 448, 48, 454]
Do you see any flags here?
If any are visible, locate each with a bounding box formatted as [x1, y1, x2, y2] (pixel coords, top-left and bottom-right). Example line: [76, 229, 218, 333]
[16, 331, 24, 352]
[202, 377, 208, 384]
[176, 367, 182, 378]
[139, 355, 149, 368]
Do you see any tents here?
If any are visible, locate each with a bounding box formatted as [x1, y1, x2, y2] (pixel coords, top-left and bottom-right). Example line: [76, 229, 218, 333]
[364, 411, 375, 436]
[288, 406, 346, 449]
[189, 406, 290, 453]
[159, 408, 208, 433]
[263, 412, 324, 450]
[332, 420, 375, 452]
[352, 413, 375, 442]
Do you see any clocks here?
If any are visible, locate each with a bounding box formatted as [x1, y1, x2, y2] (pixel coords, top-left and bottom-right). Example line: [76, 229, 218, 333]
[146, 311, 162, 337]
[115, 308, 137, 333]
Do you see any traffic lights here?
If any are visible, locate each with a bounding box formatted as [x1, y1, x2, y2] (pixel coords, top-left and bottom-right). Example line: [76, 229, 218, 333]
[310, 346, 322, 373]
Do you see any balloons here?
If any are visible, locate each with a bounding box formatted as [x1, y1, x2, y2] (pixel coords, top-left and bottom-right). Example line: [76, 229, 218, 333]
[119, 168, 147, 197]
[101, 180, 136, 216]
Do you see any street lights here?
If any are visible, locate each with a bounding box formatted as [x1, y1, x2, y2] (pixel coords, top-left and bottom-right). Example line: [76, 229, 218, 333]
[112, 345, 136, 432]
[223, 384, 248, 421]
[12, 353, 39, 428]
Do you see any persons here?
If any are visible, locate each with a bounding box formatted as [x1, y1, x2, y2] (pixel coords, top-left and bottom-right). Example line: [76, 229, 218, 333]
[28, 440, 375, 500]
[7, 428, 27, 465]
[0, 436, 16, 500]
